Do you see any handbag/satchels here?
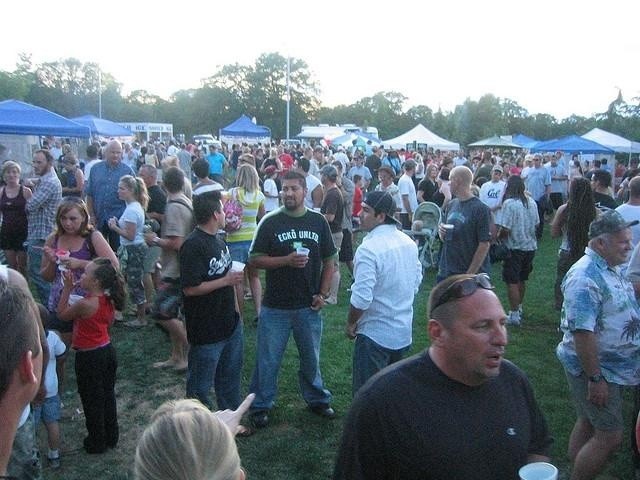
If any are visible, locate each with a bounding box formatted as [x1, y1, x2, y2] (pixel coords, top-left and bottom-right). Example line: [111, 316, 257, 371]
[223, 199, 244, 233]
[489, 241, 511, 265]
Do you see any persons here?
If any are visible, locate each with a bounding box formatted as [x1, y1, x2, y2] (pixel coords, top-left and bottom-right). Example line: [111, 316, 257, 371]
[0, 135, 640, 480]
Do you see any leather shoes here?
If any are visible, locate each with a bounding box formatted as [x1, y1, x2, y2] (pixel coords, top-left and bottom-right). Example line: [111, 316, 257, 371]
[308, 404, 335, 417]
[250, 412, 269, 428]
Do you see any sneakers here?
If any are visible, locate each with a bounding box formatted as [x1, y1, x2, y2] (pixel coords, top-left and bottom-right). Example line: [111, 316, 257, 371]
[324, 297, 337, 305]
[46, 453, 61, 469]
[115, 306, 154, 331]
[505, 307, 523, 327]
[346, 280, 354, 292]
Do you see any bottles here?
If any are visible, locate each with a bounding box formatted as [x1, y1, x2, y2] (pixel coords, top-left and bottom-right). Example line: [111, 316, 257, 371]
[144, 221, 152, 235]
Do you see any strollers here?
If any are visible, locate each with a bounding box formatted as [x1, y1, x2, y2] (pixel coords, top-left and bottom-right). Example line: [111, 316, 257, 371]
[401, 201, 442, 274]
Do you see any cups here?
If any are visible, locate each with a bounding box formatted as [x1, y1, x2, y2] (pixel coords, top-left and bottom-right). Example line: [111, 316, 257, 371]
[231, 260, 245, 271]
[297, 248, 310, 256]
[67, 294, 84, 309]
[444, 224, 454, 240]
[517, 462, 559, 479]
[56, 251, 70, 258]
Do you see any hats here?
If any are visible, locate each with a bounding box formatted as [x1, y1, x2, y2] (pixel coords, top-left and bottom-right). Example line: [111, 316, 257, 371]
[587, 209, 639, 240]
[318, 164, 338, 177]
[374, 165, 396, 179]
[362, 190, 402, 225]
[167, 146, 178, 155]
[493, 165, 504, 173]
[264, 165, 281, 175]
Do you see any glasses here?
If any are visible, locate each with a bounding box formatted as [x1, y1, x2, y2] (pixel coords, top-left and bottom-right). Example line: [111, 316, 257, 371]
[429, 273, 495, 317]
